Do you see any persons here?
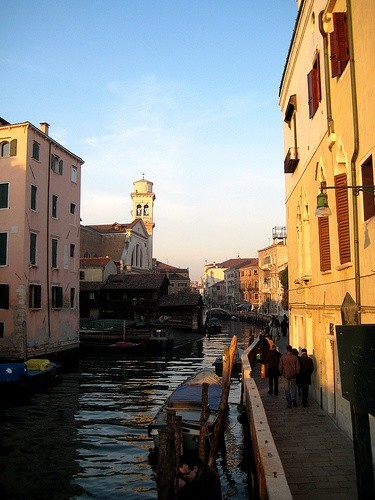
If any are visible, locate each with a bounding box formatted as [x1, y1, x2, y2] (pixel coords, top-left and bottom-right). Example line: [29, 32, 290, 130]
[271, 314, 288, 339]
[257, 336, 274, 379]
[267, 344, 282, 395]
[176, 454, 222, 500]
[279, 345, 314, 407]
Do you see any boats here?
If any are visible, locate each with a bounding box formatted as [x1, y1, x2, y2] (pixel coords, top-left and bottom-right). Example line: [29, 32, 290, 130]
[147, 369, 223, 454]
[76, 338, 148, 355]
[212, 347, 245, 373]
[0, 358, 63, 390]
[210, 324, 222, 334]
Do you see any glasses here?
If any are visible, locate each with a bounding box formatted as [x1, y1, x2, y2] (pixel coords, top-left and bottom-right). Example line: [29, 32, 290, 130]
[182, 471, 190, 475]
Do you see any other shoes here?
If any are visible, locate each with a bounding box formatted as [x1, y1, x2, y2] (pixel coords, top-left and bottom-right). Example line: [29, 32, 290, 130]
[287, 401, 297, 408]
[298, 392, 301, 397]
[302, 404, 308, 407]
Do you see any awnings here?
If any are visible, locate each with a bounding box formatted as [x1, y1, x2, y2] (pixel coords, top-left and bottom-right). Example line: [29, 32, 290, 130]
[238, 304, 251, 308]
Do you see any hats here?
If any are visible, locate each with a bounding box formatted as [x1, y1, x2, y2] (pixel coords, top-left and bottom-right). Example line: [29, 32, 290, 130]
[301, 349, 307, 352]
